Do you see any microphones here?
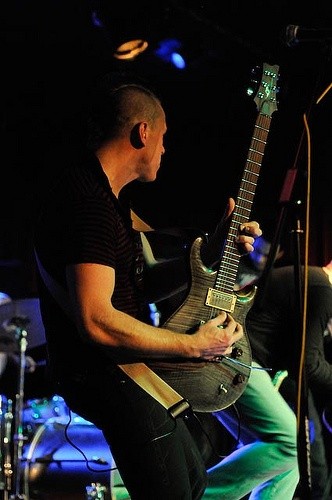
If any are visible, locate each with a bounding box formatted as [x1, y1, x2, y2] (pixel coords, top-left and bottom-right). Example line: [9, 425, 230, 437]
[2, 315, 20, 332]
[285, 24, 332, 47]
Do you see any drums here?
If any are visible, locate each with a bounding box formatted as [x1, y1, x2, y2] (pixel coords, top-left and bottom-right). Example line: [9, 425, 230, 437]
[24, 415, 131, 500]
[24, 397, 78, 443]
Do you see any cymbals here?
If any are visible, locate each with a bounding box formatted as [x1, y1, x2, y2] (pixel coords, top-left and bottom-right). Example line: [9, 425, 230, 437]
[0, 299, 47, 353]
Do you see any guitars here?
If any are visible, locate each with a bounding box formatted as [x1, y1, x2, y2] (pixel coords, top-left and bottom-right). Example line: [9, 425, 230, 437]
[150, 63, 281, 413]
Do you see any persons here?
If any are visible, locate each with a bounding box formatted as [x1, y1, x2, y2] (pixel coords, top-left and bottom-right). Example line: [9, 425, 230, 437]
[197, 212, 332, 500]
[32, 85, 263, 500]
